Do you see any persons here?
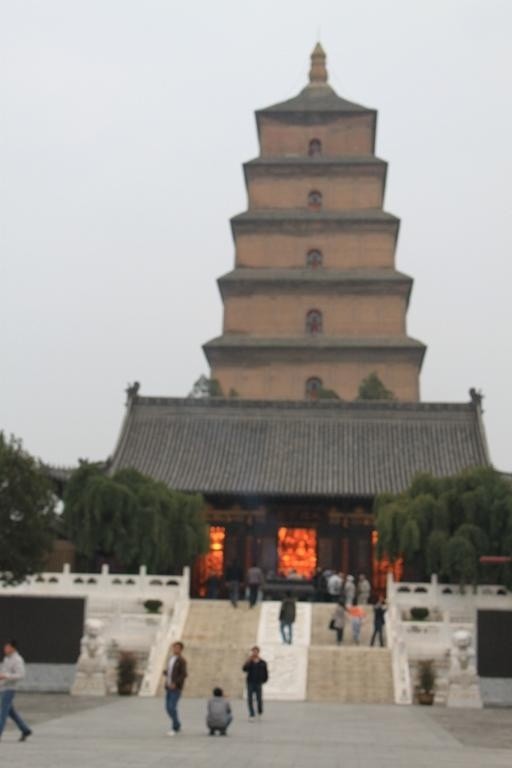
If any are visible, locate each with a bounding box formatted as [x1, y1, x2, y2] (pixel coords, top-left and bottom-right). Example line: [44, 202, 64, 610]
[0, 640, 32, 741]
[206, 688, 233, 735]
[200, 563, 389, 647]
[243, 647, 268, 717]
[163, 642, 188, 737]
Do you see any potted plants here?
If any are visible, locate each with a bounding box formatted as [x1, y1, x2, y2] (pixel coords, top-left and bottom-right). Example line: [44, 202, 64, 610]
[114, 650, 137, 696]
[412, 658, 435, 705]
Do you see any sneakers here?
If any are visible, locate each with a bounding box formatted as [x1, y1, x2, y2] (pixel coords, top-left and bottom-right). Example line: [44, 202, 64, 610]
[19, 729, 32, 741]
